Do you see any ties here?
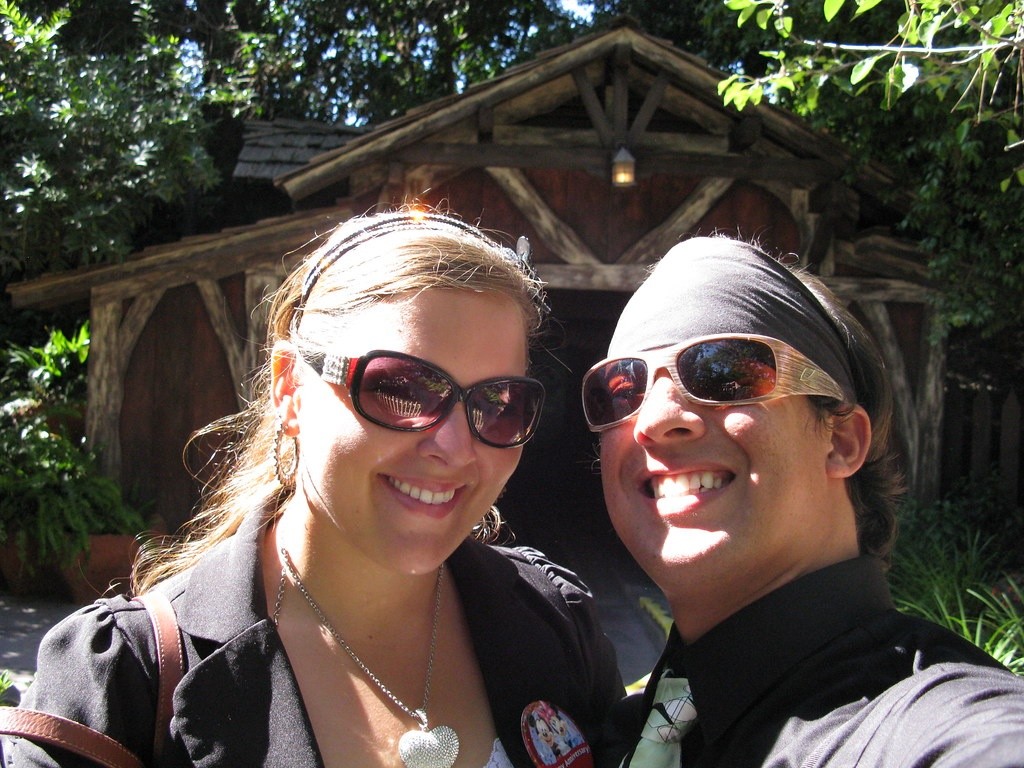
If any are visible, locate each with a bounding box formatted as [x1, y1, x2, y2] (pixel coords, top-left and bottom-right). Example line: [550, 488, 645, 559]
[618, 673, 699, 767]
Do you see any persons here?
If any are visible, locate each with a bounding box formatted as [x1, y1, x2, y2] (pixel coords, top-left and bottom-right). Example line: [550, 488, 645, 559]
[0, 207, 629, 768]
[595, 237, 1024, 768]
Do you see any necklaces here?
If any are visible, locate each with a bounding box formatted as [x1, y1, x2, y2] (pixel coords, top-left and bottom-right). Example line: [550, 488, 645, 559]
[273, 538, 460, 768]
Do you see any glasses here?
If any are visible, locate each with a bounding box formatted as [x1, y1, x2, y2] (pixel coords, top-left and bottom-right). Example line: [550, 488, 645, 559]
[581, 333, 845, 431]
[291, 350, 545, 448]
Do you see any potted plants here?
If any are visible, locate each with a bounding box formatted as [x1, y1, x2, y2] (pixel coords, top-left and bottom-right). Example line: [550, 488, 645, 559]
[0, 320, 166, 603]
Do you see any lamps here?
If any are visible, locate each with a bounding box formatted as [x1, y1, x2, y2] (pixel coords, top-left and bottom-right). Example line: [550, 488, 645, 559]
[611, 147, 636, 186]
